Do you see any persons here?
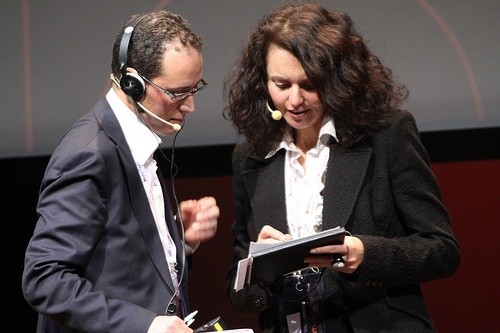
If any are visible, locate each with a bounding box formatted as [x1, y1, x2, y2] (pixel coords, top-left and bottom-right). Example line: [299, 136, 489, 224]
[18, 8, 220, 333]
[220, 0, 464, 333]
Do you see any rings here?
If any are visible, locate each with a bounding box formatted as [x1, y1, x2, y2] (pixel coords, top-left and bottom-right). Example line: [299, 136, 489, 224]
[330, 253, 347, 271]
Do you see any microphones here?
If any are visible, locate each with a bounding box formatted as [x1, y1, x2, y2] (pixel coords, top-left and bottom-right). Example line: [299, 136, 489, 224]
[135, 100, 182, 132]
[265, 102, 282, 121]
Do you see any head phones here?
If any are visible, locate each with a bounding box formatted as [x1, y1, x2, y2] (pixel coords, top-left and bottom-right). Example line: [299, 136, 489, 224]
[119, 14, 145, 99]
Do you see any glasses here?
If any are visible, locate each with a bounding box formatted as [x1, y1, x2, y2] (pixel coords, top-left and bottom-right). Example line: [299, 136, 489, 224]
[138, 73, 208, 100]
